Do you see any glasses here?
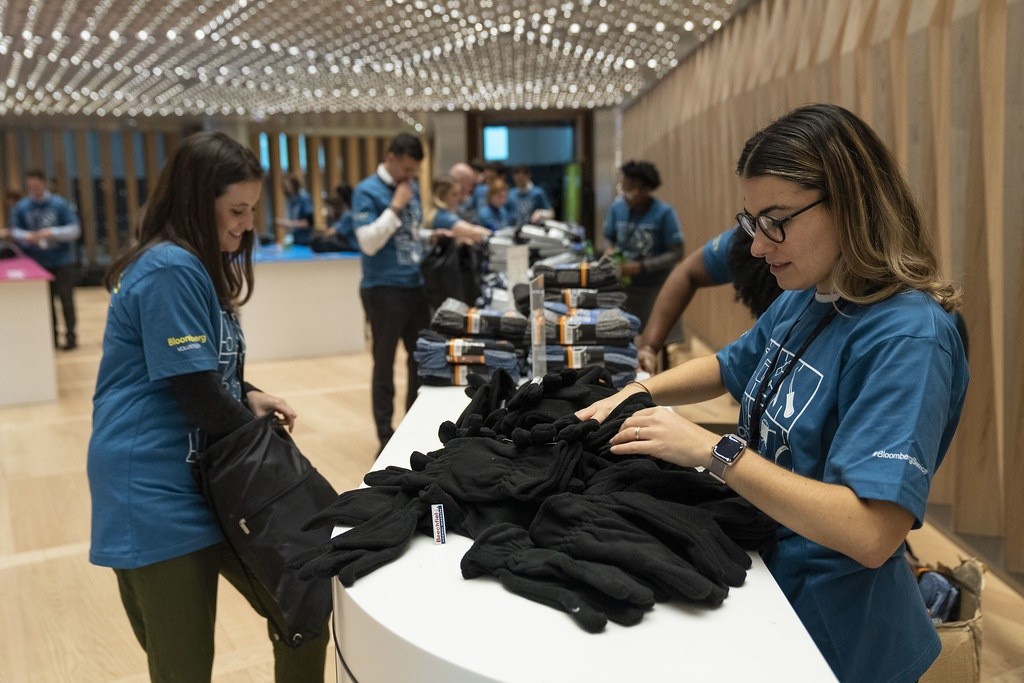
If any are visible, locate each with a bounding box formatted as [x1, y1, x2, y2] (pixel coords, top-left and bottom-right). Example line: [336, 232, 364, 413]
[734, 194, 829, 243]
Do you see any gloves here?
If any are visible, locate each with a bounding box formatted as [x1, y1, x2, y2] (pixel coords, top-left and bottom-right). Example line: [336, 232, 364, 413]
[291, 364, 767, 636]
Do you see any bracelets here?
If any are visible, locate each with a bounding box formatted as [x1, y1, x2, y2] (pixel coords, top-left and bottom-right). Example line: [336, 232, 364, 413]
[627, 381, 652, 401]
[390, 205, 399, 212]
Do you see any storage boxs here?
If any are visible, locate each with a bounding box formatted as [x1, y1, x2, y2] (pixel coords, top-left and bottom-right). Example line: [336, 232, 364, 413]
[919, 602, 983, 683]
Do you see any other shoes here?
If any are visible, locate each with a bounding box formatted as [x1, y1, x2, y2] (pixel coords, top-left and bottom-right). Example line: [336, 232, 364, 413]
[66, 334, 76, 348]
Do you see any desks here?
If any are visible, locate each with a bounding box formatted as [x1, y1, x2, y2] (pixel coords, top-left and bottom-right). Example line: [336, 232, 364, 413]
[233, 241, 365, 362]
[329, 359, 837, 683]
[0, 255, 62, 405]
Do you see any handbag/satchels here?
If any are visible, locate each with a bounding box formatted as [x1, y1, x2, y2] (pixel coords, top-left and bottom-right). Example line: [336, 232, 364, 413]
[190, 415, 339, 647]
[419, 237, 487, 307]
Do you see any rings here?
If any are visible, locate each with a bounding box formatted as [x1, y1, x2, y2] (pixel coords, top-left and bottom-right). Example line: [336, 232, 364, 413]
[635, 427, 640, 439]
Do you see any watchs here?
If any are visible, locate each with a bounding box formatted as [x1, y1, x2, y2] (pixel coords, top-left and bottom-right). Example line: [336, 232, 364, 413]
[709, 433, 748, 484]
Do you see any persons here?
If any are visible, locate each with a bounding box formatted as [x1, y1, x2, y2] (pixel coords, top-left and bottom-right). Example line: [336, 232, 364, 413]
[10, 169, 82, 350]
[574, 103, 970, 683]
[351, 132, 456, 458]
[602, 159, 684, 334]
[636, 225, 784, 376]
[277, 174, 359, 252]
[425, 161, 556, 250]
[87, 130, 334, 683]
